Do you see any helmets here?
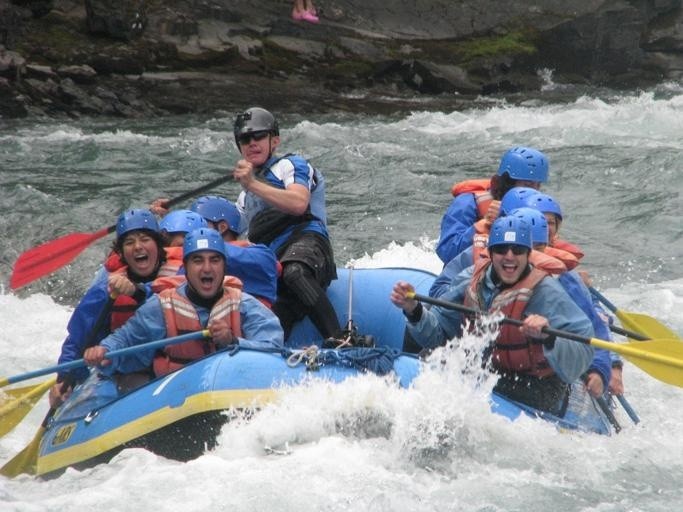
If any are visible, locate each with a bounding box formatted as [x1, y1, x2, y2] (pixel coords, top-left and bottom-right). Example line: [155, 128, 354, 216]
[488, 145, 561, 250]
[115, 196, 241, 259]
[233, 107, 277, 139]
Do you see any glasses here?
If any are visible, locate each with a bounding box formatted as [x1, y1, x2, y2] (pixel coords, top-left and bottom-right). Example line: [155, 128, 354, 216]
[240, 132, 266, 145]
[492, 243, 526, 254]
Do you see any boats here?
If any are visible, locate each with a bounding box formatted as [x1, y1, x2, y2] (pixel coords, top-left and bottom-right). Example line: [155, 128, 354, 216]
[34, 265, 612, 482]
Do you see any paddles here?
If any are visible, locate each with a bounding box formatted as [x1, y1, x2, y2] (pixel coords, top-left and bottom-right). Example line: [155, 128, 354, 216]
[0, 291, 115, 477]
[11, 163, 241, 288]
[588, 285, 678, 340]
[405, 291, 682, 389]
[0, 378, 58, 436]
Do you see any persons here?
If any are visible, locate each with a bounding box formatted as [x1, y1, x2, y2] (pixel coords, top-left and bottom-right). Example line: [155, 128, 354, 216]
[149, 107, 355, 348]
[49, 210, 187, 411]
[517, 193, 624, 395]
[389, 217, 595, 418]
[435, 146, 548, 271]
[499, 187, 543, 217]
[91, 209, 208, 245]
[189, 195, 283, 308]
[430, 207, 612, 399]
[83, 227, 285, 382]
